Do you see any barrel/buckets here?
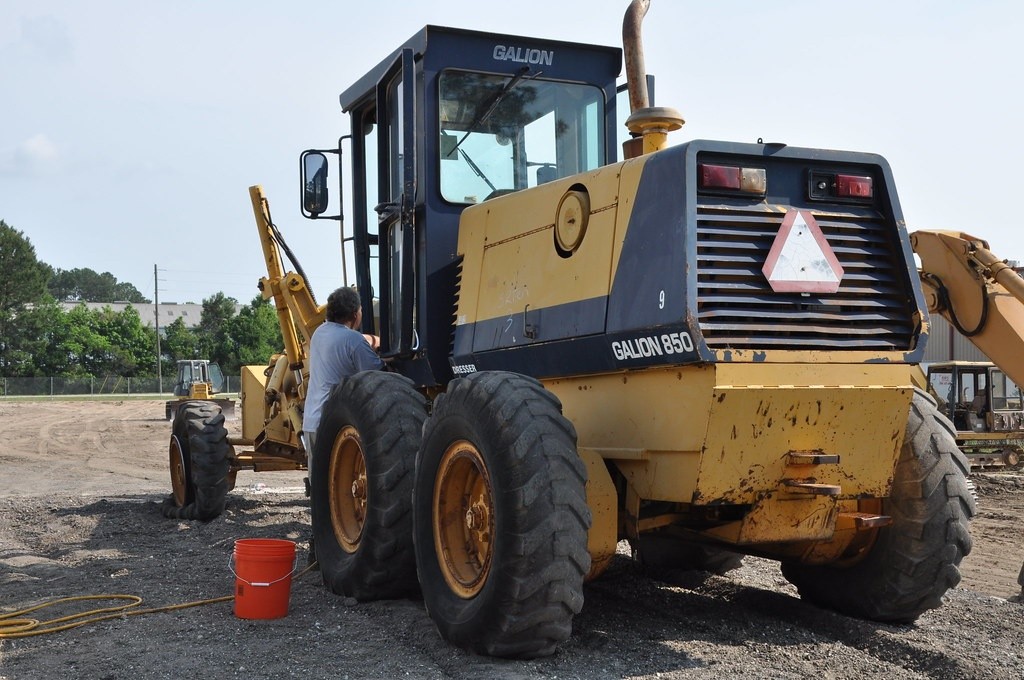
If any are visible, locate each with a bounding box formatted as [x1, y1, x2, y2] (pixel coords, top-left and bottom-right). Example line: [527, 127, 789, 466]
[228, 539, 298, 619]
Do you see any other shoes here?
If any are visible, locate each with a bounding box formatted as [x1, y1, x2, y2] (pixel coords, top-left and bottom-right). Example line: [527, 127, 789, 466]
[306, 535, 320, 571]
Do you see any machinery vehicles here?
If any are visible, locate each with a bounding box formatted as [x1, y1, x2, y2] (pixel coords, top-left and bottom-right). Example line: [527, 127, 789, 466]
[166, 0, 1024, 666]
[166, 359, 238, 422]
[925, 358, 1024, 473]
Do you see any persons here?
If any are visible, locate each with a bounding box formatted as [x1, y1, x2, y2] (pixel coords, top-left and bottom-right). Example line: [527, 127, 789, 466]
[301, 287, 389, 568]
